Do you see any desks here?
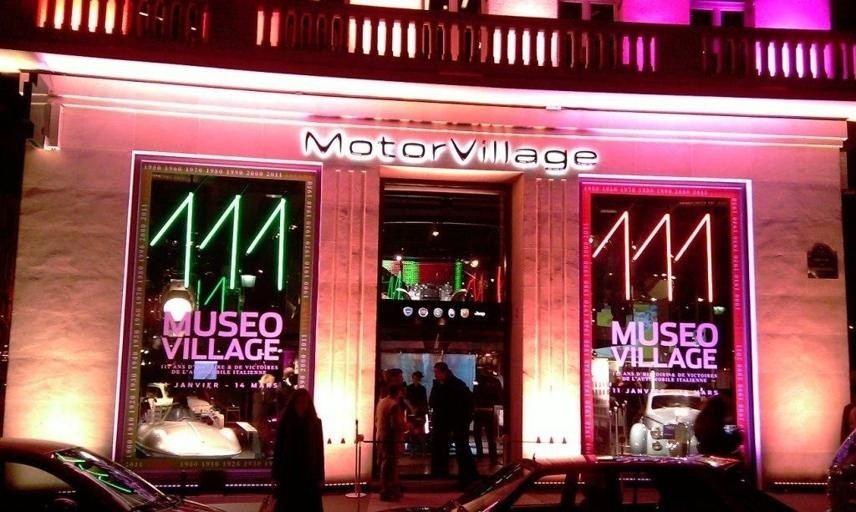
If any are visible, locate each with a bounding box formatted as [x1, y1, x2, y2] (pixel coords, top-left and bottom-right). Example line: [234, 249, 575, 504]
[224, 407, 240, 425]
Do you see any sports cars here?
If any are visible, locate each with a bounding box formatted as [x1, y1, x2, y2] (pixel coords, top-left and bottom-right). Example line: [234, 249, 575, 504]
[135, 406, 243, 459]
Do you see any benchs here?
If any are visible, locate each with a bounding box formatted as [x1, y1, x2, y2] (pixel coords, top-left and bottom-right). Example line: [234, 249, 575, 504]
[235, 420, 257, 443]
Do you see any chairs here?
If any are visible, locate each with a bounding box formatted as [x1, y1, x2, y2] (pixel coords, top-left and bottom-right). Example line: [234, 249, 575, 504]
[577, 471, 624, 509]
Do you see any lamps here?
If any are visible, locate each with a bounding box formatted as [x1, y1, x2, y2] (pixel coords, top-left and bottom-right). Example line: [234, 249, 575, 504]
[159, 278, 195, 323]
[430, 221, 440, 238]
[237, 255, 262, 288]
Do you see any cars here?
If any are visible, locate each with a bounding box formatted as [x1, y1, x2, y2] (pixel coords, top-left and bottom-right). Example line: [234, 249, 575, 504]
[379, 458, 796, 511]
[254, 397, 279, 454]
[138, 381, 214, 417]
[0, 436, 226, 512]
[630, 388, 707, 459]
[828, 429, 856, 511]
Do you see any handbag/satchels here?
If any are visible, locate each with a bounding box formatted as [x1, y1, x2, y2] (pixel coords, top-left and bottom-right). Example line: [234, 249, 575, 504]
[258, 495, 277, 512]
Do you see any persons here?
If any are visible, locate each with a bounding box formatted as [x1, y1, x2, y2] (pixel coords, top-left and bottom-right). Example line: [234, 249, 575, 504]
[275, 366, 301, 410]
[253, 373, 279, 429]
[270, 387, 326, 512]
[376, 362, 504, 501]
[692, 392, 744, 459]
